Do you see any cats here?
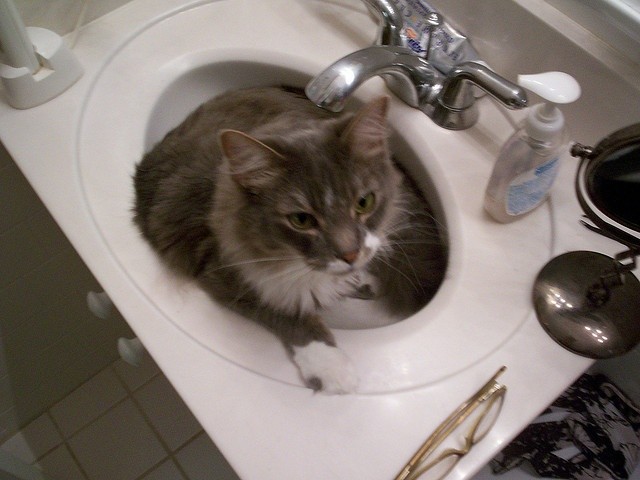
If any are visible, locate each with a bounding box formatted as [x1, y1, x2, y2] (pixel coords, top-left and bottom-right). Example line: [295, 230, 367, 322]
[130, 83, 450, 394]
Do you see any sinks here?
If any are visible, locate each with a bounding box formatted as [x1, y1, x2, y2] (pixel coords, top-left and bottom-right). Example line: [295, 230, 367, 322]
[141, 60, 451, 334]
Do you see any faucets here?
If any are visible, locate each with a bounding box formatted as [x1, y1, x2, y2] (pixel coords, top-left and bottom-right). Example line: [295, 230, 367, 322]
[304, 0, 531, 130]
